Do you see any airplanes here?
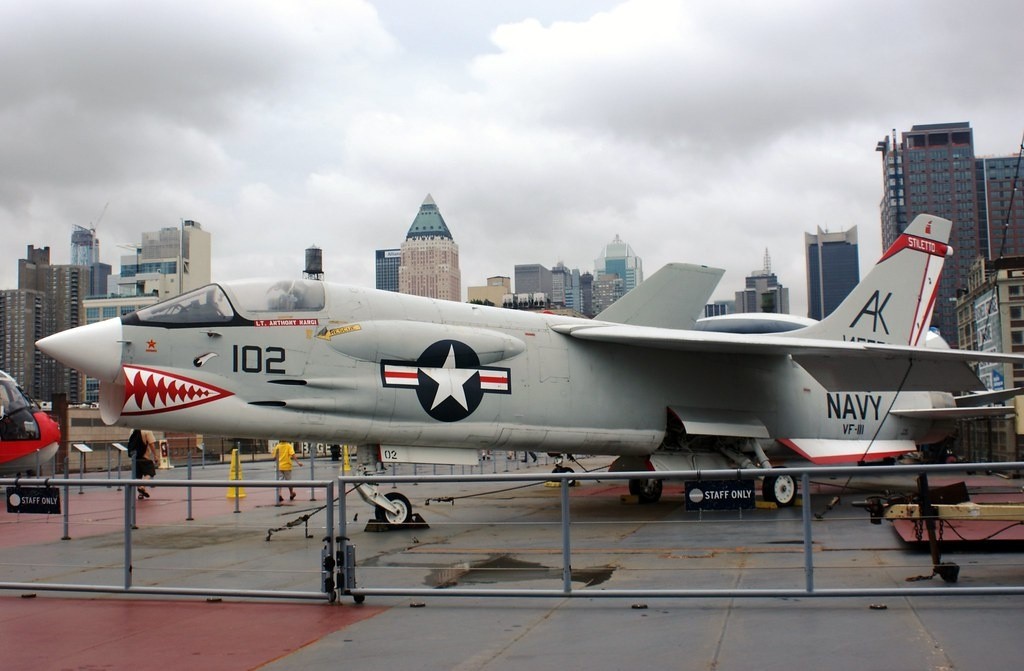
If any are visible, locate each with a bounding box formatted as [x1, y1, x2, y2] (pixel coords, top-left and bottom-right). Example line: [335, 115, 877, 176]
[34, 212, 1023, 508]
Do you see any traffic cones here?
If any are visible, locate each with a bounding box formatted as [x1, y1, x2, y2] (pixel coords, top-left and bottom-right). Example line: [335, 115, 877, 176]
[226, 447, 249, 499]
[341, 443, 353, 474]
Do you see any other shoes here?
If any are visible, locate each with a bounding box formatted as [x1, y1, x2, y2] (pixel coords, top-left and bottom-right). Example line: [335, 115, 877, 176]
[138, 485, 150, 498]
[279, 496, 284, 502]
[289, 492, 296, 500]
[137, 493, 144, 500]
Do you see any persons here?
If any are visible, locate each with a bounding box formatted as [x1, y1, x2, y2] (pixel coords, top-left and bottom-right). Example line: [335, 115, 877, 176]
[130, 429, 158, 499]
[331, 444, 340, 462]
[272, 440, 303, 500]
[480, 446, 572, 463]
